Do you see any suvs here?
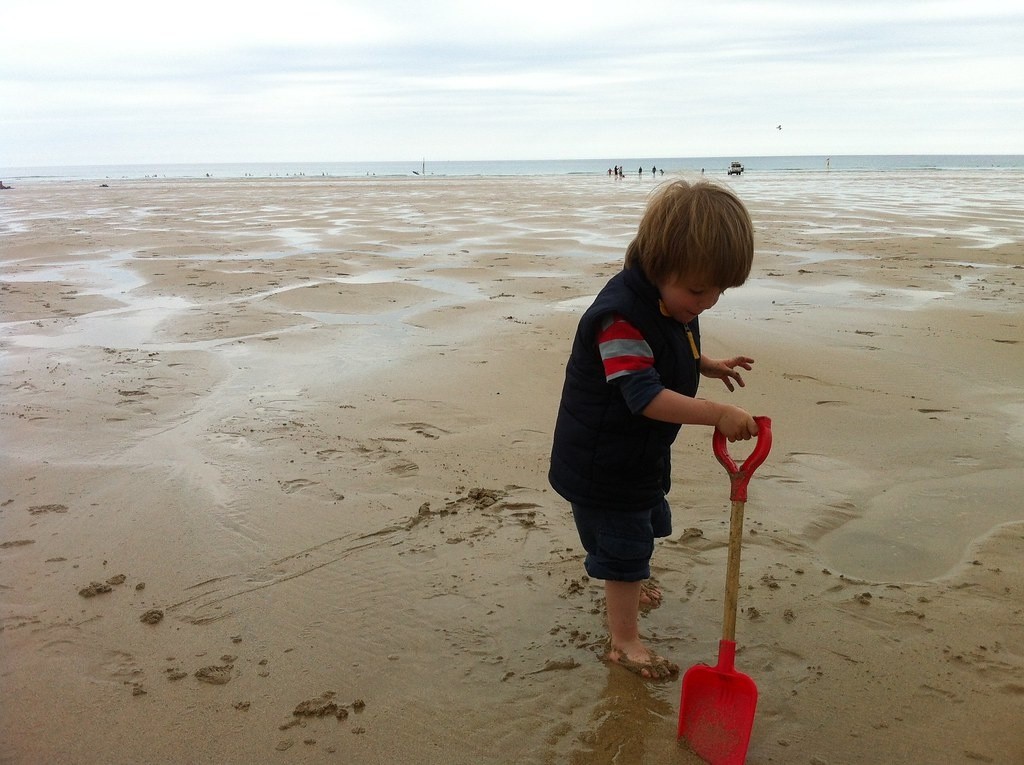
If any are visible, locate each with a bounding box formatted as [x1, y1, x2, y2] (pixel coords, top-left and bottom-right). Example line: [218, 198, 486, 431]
[727, 161, 744, 176]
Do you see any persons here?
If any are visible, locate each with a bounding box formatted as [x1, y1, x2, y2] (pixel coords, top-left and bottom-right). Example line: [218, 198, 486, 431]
[608, 165, 622, 177]
[549, 178, 755, 678]
[660, 169, 664, 176]
[652, 166, 656, 174]
[638, 166, 642, 174]
